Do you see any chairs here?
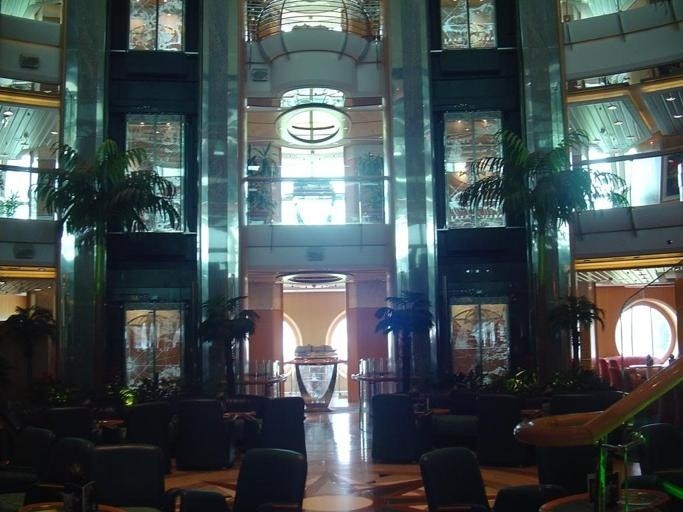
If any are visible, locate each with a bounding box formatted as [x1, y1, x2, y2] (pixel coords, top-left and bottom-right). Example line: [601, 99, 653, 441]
[354, 388, 681, 512]
[1, 390, 308, 511]
